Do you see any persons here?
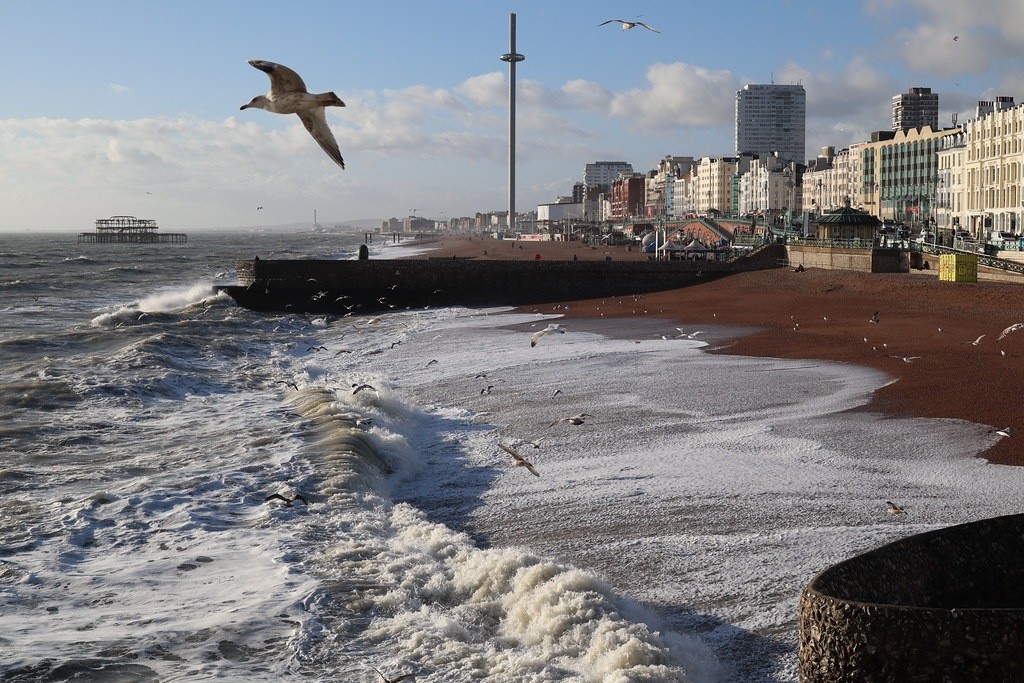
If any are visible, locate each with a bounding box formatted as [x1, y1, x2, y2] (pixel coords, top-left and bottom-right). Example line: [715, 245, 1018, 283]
[483, 250, 487, 255]
[870, 311, 879, 323]
[939, 234, 943, 243]
[519, 245, 523, 250]
[511, 241, 515, 248]
[573, 254, 577, 261]
[918, 260, 929, 270]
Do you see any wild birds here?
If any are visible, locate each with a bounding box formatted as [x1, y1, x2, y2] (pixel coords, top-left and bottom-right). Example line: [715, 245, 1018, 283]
[865, 311, 879, 324]
[1000, 350, 1007, 356]
[823, 316, 826, 321]
[32, 296, 40, 302]
[790, 315, 801, 331]
[595, 293, 718, 320]
[961, 334, 986, 346]
[884, 354, 921, 365]
[239, 13, 662, 172]
[71, 191, 242, 332]
[863, 336, 888, 351]
[937, 327, 944, 333]
[996, 323, 1024, 342]
[530, 323, 570, 348]
[263, 494, 307, 508]
[473, 373, 597, 478]
[661, 327, 709, 340]
[532, 304, 569, 314]
[273, 286, 445, 429]
[880, 420, 1015, 528]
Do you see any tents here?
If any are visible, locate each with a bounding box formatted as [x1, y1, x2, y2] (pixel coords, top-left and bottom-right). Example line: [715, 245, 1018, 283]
[657, 239, 682, 261]
[682, 239, 708, 260]
